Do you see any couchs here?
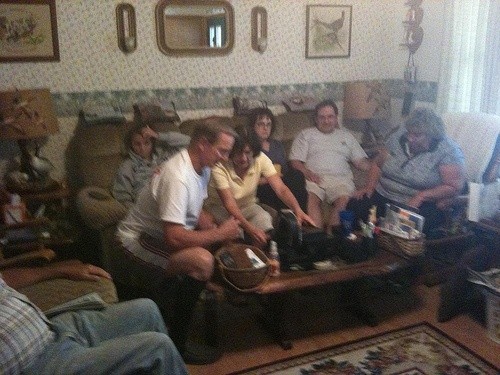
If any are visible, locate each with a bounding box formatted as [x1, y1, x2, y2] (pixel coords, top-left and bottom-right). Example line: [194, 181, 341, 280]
[72, 111, 368, 266]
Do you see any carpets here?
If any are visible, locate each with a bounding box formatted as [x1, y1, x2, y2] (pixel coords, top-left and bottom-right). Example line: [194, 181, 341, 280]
[225, 320, 499, 375]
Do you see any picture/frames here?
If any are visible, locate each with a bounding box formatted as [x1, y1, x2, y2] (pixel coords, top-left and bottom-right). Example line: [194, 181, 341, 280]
[0, 0, 61, 63]
[305, 5, 352, 59]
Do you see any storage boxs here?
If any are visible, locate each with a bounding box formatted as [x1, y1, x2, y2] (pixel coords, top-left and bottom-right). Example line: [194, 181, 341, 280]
[467, 268, 499, 341]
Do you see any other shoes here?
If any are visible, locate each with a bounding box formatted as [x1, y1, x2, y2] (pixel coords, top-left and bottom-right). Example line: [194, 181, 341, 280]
[175, 336, 222, 365]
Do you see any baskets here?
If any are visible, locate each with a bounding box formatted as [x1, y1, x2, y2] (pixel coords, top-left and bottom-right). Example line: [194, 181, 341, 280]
[373, 222, 426, 260]
[214, 244, 272, 292]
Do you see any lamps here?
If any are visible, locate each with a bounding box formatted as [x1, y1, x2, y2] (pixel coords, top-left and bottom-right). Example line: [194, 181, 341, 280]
[342, 81, 393, 148]
[0, 86, 58, 195]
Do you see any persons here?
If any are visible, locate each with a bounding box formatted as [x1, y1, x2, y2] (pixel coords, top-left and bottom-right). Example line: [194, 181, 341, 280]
[0, 264, 189, 375]
[112, 100, 465, 364]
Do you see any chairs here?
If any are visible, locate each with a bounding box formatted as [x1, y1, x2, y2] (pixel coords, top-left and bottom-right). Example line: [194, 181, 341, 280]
[420, 109, 500, 247]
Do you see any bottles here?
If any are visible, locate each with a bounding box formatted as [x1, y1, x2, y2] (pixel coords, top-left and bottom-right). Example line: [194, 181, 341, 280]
[368, 227, 376, 255]
[270, 241, 280, 276]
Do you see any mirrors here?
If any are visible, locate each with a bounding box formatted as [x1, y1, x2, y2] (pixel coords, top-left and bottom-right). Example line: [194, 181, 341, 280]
[155, 0, 235, 57]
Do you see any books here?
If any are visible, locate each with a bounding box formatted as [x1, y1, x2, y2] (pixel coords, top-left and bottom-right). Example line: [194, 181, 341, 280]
[43, 293, 109, 316]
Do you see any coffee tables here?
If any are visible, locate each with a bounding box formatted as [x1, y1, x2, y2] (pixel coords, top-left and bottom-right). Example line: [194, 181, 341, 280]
[250, 248, 424, 350]
